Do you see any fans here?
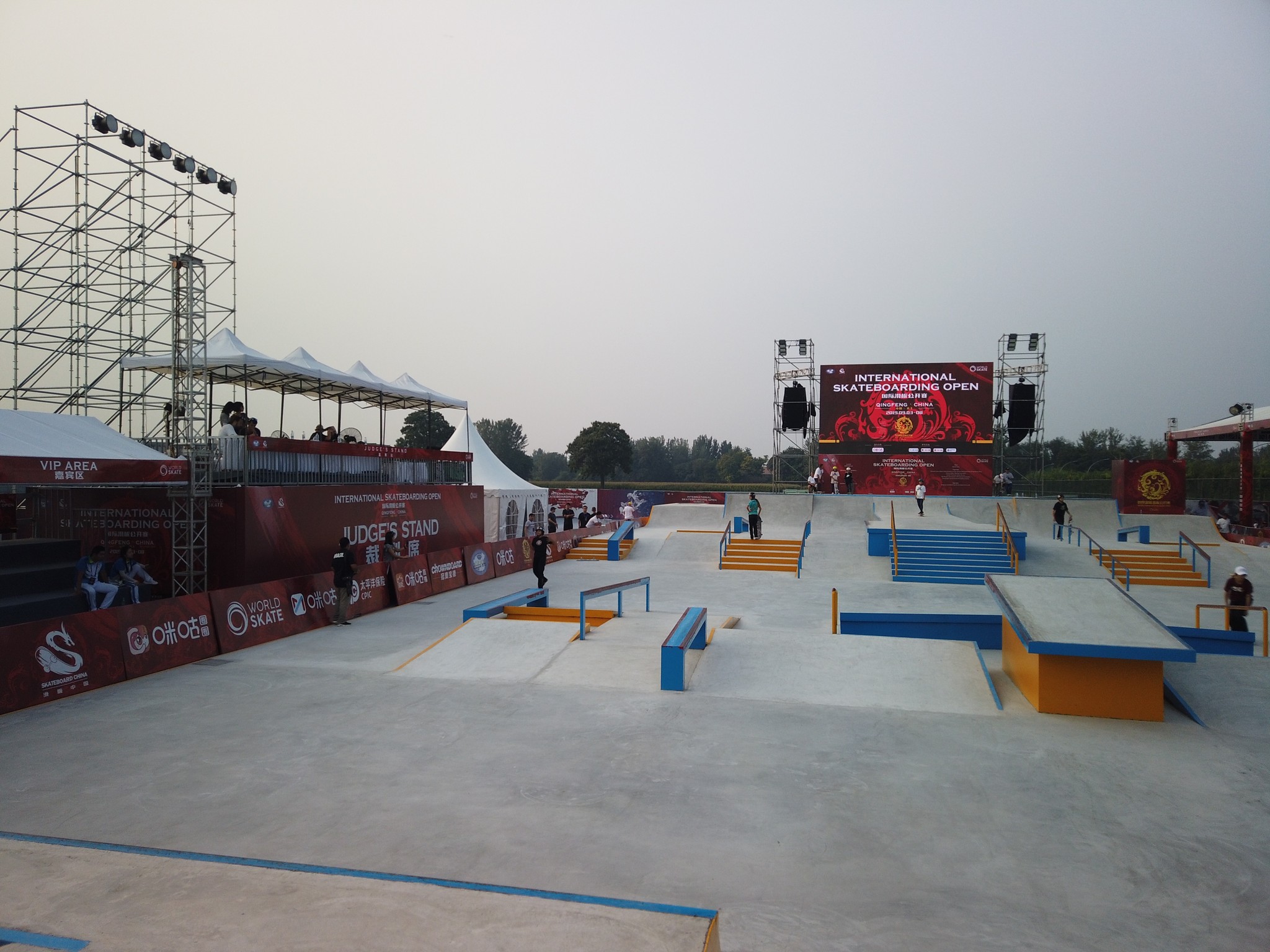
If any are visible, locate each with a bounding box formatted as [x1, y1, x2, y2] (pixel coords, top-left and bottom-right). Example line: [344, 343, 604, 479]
[271, 430, 291, 439]
[340, 427, 362, 444]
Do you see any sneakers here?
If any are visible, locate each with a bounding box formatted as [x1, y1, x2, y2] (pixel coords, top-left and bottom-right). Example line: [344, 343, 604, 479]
[332, 620, 351, 626]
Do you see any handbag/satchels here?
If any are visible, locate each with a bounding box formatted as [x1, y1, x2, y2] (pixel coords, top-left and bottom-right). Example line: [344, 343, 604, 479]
[107, 576, 126, 587]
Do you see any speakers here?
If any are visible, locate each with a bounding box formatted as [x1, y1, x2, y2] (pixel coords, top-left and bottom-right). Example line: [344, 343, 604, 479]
[782, 387, 807, 430]
[1008, 384, 1036, 429]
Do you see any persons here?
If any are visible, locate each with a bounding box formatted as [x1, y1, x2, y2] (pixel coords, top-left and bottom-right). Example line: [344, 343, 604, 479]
[309, 424, 338, 443]
[331, 537, 358, 626]
[217, 400, 260, 471]
[524, 513, 536, 537]
[74, 545, 119, 611]
[993, 474, 1004, 497]
[1224, 566, 1254, 633]
[578, 506, 591, 528]
[109, 546, 158, 603]
[623, 502, 635, 521]
[807, 472, 816, 493]
[590, 507, 597, 518]
[1052, 494, 1072, 541]
[830, 466, 840, 495]
[526, 526, 557, 589]
[814, 464, 824, 494]
[382, 531, 410, 602]
[547, 507, 558, 533]
[562, 503, 575, 531]
[1002, 467, 1015, 496]
[585, 511, 602, 528]
[1216, 513, 1231, 541]
[844, 467, 854, 494]
[746, 492, 761, 540]
[915, 479, 926, 514]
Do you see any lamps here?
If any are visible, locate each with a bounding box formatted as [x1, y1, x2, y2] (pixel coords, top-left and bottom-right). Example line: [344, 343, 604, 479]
[778, 339, 787, 355]
[173, 153, 195, 173]
[119, 126, 145, 148]
[1007, 334, 1017, 351]
[92, 112, 118, 134]
[799, 339, 807, 356]
[196, 165, 217, 184]
[218, 176, 237, 195]
[148, 139, 172, 160]
[1229, 404, 1244, 415]
[1029, 334, 1038, 351]
[163, 403, 172, 413]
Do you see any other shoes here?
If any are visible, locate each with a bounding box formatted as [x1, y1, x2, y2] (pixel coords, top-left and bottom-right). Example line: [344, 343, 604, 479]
[144, 577, 158, 584]
[751, 538, 754, 540]
[838, 493, 840, 495]
[542, 577, 547, 586]
[1057, 537, 1064, 540]
[755, 537, 758, 539]
[918, 511, 923, 514]
[832, 492, 834, 494]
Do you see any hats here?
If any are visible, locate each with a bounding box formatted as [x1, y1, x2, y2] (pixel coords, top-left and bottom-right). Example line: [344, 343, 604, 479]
[535, 526, 543, 530]
[551, 507, 556, 511]
[1006, 468, 1009, 469]
[1234, 566, 1247, 575]
[749, 492, 756, 499]
[316, 425, 323, 428]
[1000, 474, 1003, 478]
[1058, 494, 1064, 499]
[628, 502, 631, 504]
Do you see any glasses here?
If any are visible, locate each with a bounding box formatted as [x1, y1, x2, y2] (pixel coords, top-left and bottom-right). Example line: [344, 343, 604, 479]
[250, 418, 257, 421]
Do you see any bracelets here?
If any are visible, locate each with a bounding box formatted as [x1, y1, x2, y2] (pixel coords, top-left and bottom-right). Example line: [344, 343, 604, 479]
[404, 546, 407, 549]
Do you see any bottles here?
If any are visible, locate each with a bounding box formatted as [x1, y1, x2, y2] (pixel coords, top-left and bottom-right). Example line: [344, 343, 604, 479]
[315, 433, 319, 441]
[121, 597, 125, 606]
[1035, 493, 1037, 498]
[1015, 492, 1019, 497]
[364, 437, 367, 445]
[1021, 492, 1024, 497]
[302, 431, 305, 440]
[337, 435, 341, 443]
[291, 430, 294, 439]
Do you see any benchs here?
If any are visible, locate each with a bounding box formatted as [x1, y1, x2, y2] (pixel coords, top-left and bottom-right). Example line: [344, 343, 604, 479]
[0, 559, 152, 629]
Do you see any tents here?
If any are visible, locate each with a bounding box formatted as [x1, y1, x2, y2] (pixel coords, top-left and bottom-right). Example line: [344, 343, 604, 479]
[119, 327, 469, 484]
[439, 412, 549, 543]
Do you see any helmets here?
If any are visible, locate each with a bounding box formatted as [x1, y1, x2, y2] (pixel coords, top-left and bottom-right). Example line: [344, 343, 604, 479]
[918, 479, 923, 481]
[833, 466, 837, 469]
[846, 467, 851, 470]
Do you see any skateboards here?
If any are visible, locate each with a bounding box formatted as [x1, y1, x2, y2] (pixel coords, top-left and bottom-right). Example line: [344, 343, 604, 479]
[834, 480, 839, 495]
[918, 513, 925, 517]
[851, 480, 854, 495]
[1068, 515, 1074, 537]
[576, 557, 600, 561]
[756, 515, 763, 539]
[995, 488, 1004, 497]
[812, 491, 825, 494]
